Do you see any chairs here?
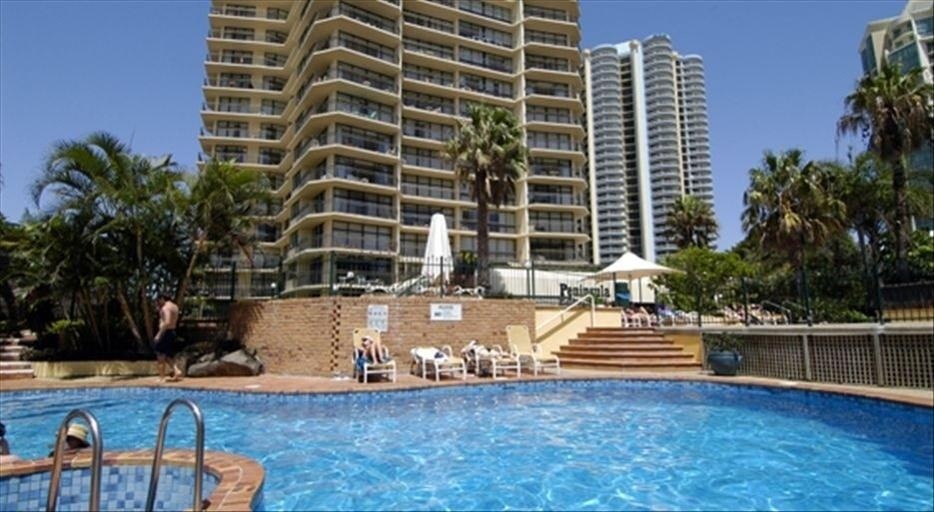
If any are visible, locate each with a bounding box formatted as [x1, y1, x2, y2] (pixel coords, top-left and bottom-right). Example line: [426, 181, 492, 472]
[462, 344, 521, 378]
[505, 325, 559, 376]
[351, 328, 396, 383]
[620, 304, 788, 328]
[409, 343, 466, 381]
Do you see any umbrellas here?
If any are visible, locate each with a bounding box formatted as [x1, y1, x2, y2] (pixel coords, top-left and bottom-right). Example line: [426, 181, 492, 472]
[419, 213, 454, 285]
[585, 248, 687, 301]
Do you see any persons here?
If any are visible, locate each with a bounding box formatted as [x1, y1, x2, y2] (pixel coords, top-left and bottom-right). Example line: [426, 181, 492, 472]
[148, 297, 172, 383]
[356, 334, 389, 364]
[49, 425, 89, 454]
[609, 302, 680, 326]
[0, 421, 10, 454]
[153, 291, 190, 385]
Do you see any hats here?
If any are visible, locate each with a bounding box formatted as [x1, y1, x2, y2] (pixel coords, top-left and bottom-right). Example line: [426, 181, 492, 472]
[361, 334, 374, 342]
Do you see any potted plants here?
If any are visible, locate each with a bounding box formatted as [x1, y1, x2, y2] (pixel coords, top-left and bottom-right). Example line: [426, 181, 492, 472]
[701, 330, 744, 375]
